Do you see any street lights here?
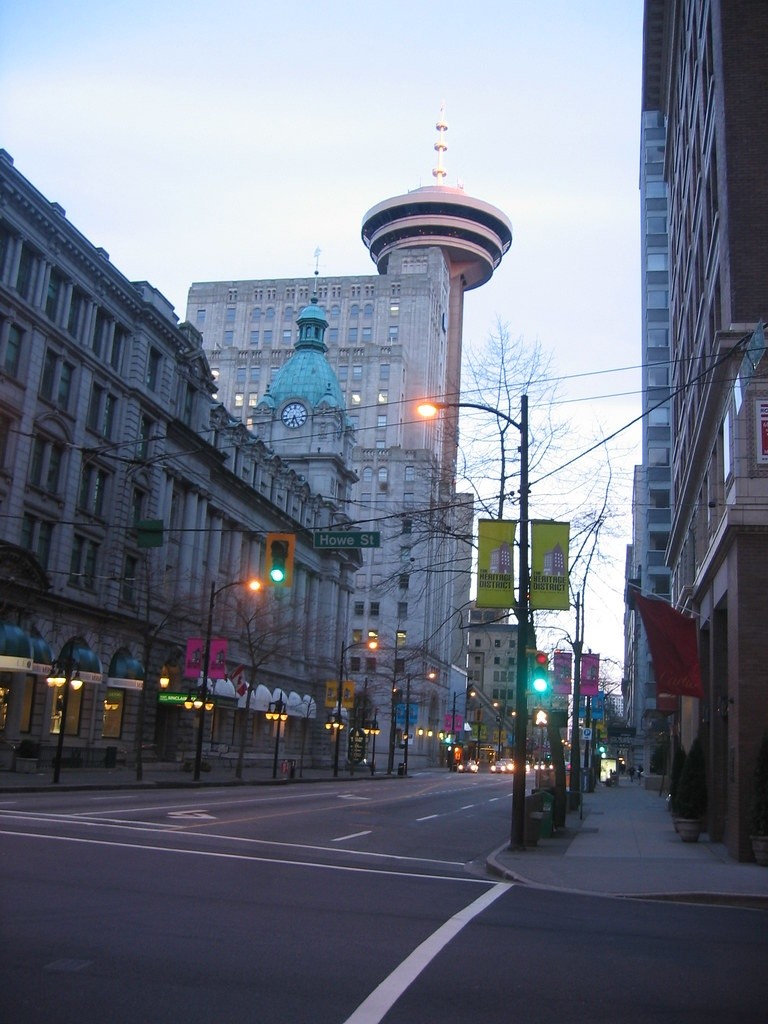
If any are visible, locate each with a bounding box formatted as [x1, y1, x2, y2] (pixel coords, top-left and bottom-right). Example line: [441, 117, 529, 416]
[438, 690, 475, 772]
[418, 393, 529, 854]
[510, 710, 517, 759]
[477, 701, 499, 762]
[42, 640, 85, 785]
[363, 708, 381, 776]
[181, 581, 261, 784]
[265, 689, 288, 779]
[322, 638, 380, 780]
[403, 673, 435, 774]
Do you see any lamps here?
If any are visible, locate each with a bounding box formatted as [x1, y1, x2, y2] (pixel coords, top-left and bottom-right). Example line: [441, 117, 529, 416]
[720, 696, 733, 721]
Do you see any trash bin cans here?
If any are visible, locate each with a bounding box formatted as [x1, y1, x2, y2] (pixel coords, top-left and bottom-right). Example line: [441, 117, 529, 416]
[105, 746, 117, 768]
[533, 791, 554, 839]
[285, 760, 296, 778]
[398, 763, 407, 775]
[524, 796, 545, 848]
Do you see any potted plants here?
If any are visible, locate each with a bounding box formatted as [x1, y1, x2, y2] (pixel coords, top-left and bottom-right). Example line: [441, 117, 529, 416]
[669, 732, 708, 842]
[14, 739, 39, 773]
[749, 733, 768, 865]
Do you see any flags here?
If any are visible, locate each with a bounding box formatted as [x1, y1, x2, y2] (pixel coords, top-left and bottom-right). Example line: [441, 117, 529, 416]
[632, 589, 705, 698]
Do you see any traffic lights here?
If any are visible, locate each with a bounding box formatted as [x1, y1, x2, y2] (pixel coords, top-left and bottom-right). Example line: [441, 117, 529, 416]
[530, 651, 547, 696]
[262, 533, 294, 588]
[531, 707, 568, 731]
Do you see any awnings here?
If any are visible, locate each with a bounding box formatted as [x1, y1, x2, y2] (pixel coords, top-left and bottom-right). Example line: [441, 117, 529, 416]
[332, 703, 347, 716]
[0, 621, 54, 674]
[107, 651, 144, 690]
[200, 672, 317, 718]
[55, 643, 103, 684]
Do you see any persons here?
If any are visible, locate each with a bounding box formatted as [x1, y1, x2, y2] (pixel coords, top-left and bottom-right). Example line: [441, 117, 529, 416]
[619, 764, 624, 774]
[637, 765, 644, 785]
[629, 766, 636, 782]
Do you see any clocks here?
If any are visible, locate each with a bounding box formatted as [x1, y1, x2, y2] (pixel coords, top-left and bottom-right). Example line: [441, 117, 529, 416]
[281, 402, 307, 428]
[338, 415, 342, 438]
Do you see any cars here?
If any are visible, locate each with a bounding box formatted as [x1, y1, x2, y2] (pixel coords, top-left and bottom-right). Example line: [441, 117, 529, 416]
[455, 758, 551, 773]
